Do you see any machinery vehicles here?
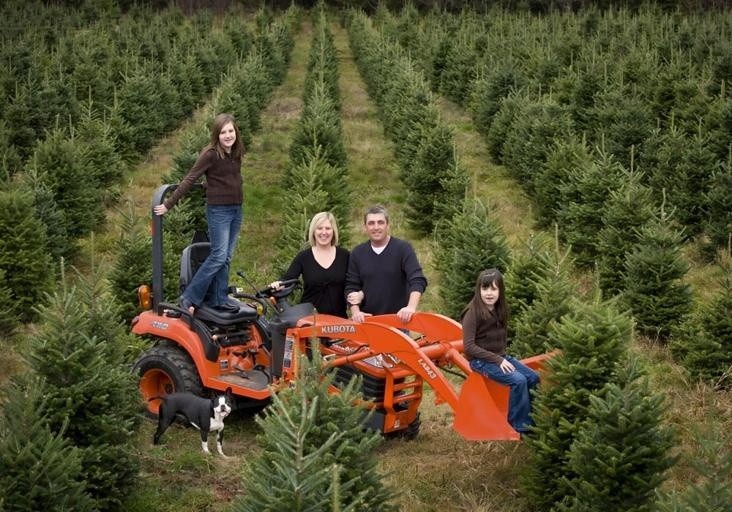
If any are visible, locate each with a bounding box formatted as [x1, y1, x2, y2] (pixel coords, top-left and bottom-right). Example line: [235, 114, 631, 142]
[130, 183, 563, 441]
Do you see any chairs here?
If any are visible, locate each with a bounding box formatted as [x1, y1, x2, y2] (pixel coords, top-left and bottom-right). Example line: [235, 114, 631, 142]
[180, 241, 258, 327]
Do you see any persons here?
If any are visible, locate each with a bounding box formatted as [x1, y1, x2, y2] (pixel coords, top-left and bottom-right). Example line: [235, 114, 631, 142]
[460, 268, 543, 442]
[153, 112, 244, 314]
[343, 203, 428, 405]
[268, 211, 365, 321]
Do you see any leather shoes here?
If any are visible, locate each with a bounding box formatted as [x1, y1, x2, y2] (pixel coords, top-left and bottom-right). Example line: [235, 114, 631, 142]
[177, 296, 192, 310]
[213, 302, 240, 312]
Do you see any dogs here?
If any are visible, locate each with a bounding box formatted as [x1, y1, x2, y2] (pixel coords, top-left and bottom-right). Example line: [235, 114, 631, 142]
[148, 387, 233, 457]
